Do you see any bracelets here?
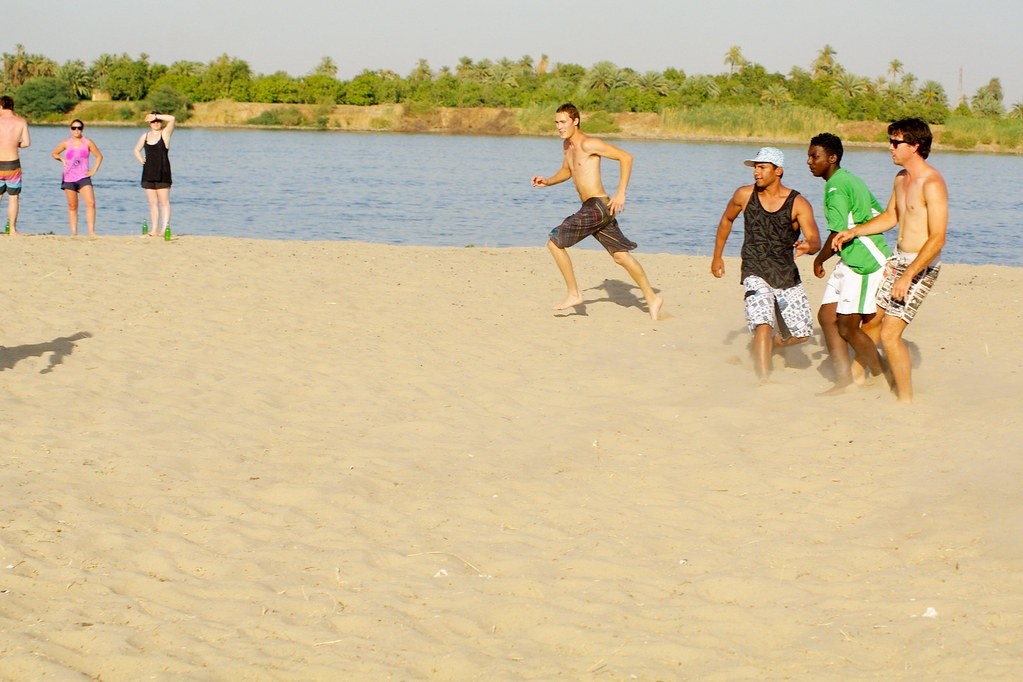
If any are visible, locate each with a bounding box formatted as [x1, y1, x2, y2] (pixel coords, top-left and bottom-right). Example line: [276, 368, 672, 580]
[155, 113, 157, 120]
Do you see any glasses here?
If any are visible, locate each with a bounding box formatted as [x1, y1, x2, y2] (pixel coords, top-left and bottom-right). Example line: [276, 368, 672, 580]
[889, 138, 906, 149]
[71, 126, 82, 130]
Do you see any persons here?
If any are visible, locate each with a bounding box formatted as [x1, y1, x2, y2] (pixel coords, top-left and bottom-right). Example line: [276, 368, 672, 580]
[134, 110, 176, 237]
[0, 95, 30, 237]
[807, 132, 895, 399]
[711, 147, 822, 382]
[831, 116, 948, 407]
[51, 119, 103, 241]
[531, 102, 664, 321]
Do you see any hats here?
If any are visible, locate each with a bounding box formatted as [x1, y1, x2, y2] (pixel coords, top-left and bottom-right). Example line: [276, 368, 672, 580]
[744, 147, 784, 167]
